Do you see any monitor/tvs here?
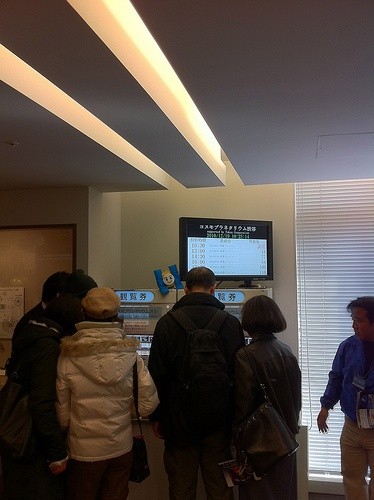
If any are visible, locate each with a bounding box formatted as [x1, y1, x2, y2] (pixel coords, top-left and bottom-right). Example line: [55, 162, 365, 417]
[176, 217, 273, 289]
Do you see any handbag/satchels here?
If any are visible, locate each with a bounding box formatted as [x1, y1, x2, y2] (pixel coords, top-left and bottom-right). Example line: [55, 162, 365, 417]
[0, 379, 33, 458]
[127, 435, 150, 482]
[235, 348, 300, 473]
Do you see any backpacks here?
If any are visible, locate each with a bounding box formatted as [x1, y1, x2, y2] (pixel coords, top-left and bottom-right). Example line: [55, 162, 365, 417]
[167, 305, 227, 394]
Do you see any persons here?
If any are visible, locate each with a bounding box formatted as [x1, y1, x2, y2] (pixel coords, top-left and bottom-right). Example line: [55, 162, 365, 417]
[56, 287, 161, 500]
[318, 296, 374, 500]
[11, 271, 97, 500]
[230, 296, 302, 500]
[147, 269, 243, 500]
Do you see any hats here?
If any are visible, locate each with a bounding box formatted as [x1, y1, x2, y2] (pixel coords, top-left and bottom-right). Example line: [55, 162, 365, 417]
[82, 287, 119, 317]
